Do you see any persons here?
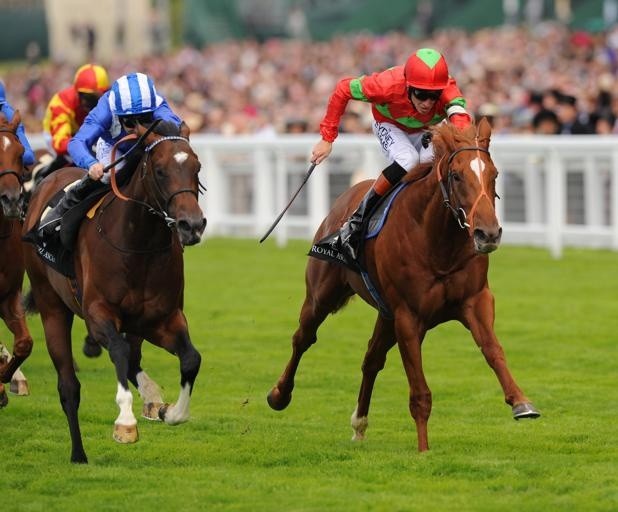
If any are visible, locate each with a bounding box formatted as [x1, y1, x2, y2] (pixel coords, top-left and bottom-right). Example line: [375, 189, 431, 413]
[36, 72, 191, 242]
[0, 82, 36, 166]
[310, 48, 471, 263]
[43, 64, 112, 177]
[0, 19, 618, 134]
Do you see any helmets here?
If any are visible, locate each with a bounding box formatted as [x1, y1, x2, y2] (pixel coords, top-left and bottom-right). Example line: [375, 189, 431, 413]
[108, 73, 157, 115]
[74, 64, 110, 94]
[406, 48, 448, 90]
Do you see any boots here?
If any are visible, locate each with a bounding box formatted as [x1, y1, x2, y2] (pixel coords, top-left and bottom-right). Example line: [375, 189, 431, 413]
[43, 172, 102, 235]
[340, 187, 381, 260]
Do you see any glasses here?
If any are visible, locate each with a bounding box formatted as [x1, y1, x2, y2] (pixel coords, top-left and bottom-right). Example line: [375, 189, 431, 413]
[121, 114, 153, 127]
[413, 87, 440, 101]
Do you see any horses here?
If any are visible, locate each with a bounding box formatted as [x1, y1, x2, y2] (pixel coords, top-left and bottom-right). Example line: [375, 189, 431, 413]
[266, 116, 541, 454]
[0, 109, 34, 409]
[20, 120, 207, 465]
